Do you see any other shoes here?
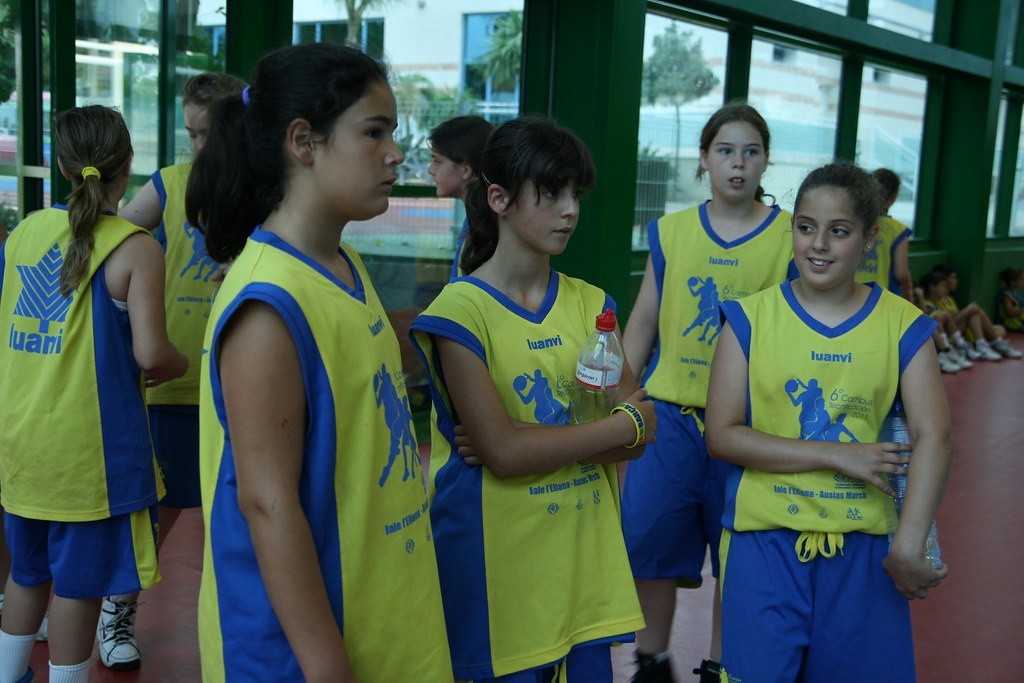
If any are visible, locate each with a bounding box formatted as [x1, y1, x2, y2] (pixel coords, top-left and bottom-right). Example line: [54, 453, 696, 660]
[973, 340, 1002, 360]
[957, 342, 982, 359]
[936, 351, 960, 373]
[629, 649, 675, 683]
[947, 349, 972, 369]
[990, 337, 1021, 358]
[692, 658, 725, 683]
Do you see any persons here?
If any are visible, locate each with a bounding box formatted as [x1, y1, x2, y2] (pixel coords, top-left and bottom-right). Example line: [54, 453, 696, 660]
[994, 269, 1024, 332]
[406, 120, 657, 683]
[430, 111, 499, 283]
[911, 262, 1022, 373]
[620, 109, 795, 683]
[185, 43, 461, 683]
[97, 76, 249, 671]
[0, 107, 188, 683]
[851, 169, 915, 302]
[705, 165, 953, 683]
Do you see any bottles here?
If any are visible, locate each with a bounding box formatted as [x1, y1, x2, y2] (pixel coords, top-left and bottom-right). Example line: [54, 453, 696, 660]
[568, 309, 624, 424]
[880, 400, 941, 569]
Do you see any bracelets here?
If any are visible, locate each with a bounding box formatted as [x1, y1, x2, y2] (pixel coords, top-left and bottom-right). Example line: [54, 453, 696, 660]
[610, 403, 647, 449]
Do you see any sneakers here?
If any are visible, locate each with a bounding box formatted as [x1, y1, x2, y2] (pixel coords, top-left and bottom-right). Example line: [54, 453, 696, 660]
[36, 613, 49, 642]
[95, 596, 141, 670]
